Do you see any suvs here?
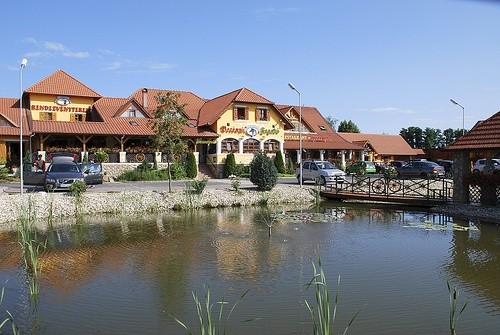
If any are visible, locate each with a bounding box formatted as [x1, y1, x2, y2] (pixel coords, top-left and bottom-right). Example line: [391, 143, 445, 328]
[472, 158, 500, 174]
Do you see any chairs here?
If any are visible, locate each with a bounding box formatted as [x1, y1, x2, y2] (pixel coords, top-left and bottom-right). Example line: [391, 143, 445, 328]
[318, 165, 322, 169]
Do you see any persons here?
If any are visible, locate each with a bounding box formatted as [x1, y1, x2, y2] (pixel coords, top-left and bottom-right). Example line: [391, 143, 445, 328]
[90, 158, 95, 189]
[35, 154, 45, 172]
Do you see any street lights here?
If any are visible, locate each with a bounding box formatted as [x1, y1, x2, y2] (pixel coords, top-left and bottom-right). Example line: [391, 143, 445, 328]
[449, 98, 464, 137]
[18, 58, 28, 195]
[288, 82, 303, 189]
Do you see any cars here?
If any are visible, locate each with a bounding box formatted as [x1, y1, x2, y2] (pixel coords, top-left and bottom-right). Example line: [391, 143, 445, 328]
[23, 153, 103, 193]
[296, 160, 346, 186]
[372, 159, 453, 181]
[345, 160, 377, 175]
